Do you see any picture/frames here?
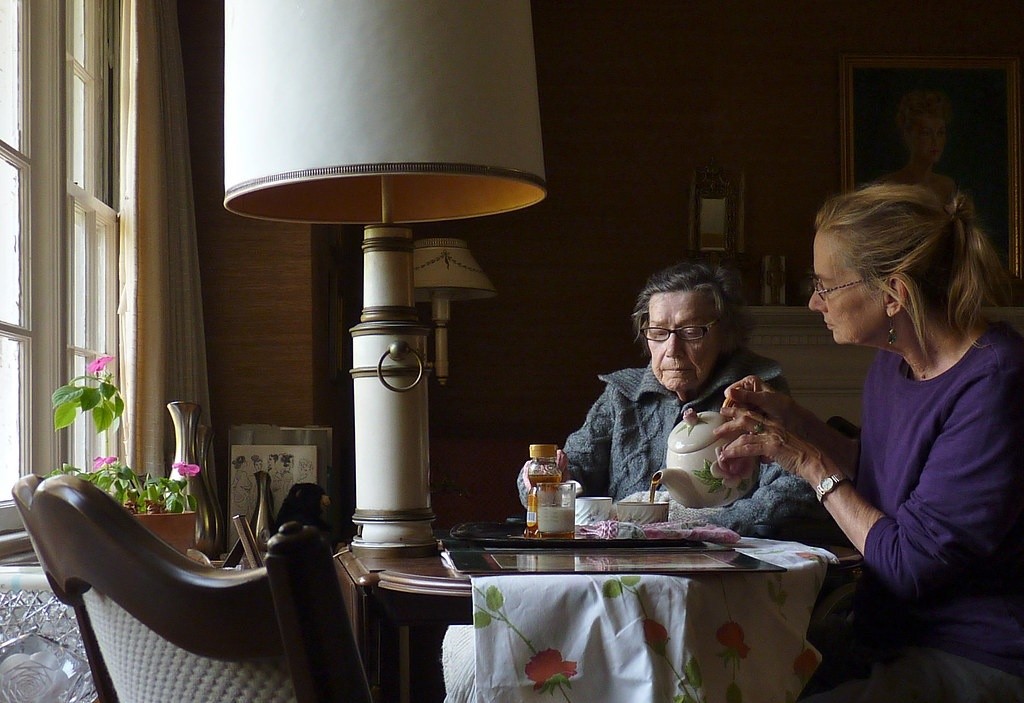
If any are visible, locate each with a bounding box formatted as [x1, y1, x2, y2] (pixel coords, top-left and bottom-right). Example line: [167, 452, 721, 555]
[838, 51, 1024, 296]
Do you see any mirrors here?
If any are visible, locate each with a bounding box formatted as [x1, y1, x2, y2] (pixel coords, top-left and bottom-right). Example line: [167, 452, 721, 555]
[689, 156, 745, 254]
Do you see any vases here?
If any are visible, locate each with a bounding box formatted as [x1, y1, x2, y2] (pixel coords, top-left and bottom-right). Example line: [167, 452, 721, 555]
[135, 512, 196, 555]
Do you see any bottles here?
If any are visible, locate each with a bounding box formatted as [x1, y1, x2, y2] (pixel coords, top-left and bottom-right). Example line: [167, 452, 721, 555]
[248, 471, 275, 552]
[522, 444, 561, 538]
[164, 402, 224, 560]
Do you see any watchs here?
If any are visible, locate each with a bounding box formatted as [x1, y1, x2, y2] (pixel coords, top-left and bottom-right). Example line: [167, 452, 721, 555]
[816, 472, 849, 503]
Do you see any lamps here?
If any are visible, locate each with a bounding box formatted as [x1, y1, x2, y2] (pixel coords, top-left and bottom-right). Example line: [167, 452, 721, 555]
[221, 0, 546, 563]
[412, 238, 498, 385]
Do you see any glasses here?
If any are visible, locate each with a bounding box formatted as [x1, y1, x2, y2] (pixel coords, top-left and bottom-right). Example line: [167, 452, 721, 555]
[813, 275, 882, 302]
[640, 316, 719, 341]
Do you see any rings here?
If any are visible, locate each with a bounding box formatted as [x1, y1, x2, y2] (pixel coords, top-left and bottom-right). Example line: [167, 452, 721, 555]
[753, 422, 765, 433]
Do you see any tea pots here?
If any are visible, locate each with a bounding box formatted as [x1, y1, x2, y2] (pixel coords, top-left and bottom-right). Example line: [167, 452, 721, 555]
[651, 386, 761, 508]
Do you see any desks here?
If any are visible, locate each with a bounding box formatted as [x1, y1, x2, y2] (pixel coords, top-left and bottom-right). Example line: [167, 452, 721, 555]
[335, 529, 866, 703]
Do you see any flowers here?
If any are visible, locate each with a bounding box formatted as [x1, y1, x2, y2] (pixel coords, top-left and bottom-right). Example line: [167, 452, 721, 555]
[37, 356, 200, 513]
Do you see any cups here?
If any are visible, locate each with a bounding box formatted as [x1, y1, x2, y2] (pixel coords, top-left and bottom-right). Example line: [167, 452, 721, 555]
[537, 483, 576, 540]
[616, 501, 669, 524]
[575, 497, 612, 526]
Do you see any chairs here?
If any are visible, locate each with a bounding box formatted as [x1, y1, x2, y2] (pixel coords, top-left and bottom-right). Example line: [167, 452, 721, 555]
[13, 472, 374, 703]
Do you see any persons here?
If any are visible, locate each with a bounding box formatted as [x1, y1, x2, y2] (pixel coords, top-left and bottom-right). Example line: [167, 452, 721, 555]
[713, 183, 1024, 703]
[516, 264, 828, 538]
